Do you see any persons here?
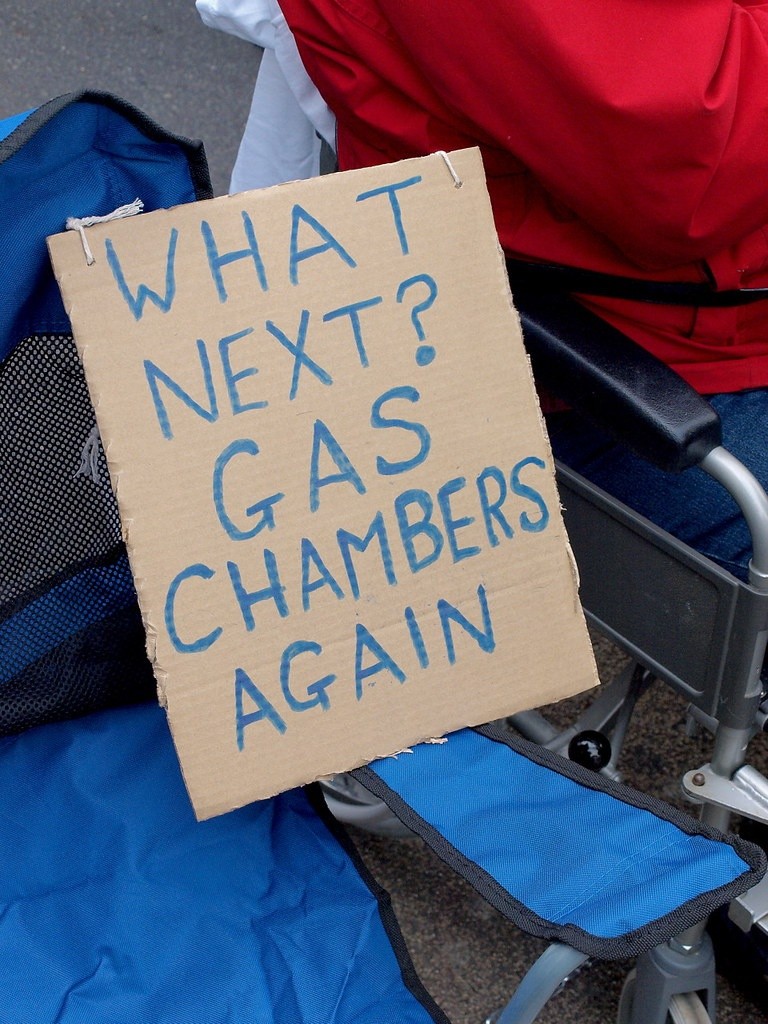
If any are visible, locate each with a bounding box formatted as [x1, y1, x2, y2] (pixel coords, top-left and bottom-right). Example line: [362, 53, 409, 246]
[276, 1, 768, 575]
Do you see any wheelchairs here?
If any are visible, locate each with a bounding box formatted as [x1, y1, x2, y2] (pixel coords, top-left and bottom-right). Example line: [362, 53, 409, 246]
[302, 135, 766, 1024]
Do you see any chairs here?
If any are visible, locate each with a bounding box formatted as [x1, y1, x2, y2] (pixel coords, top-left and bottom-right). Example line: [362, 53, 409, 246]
[1, 86, 768, 1024]
